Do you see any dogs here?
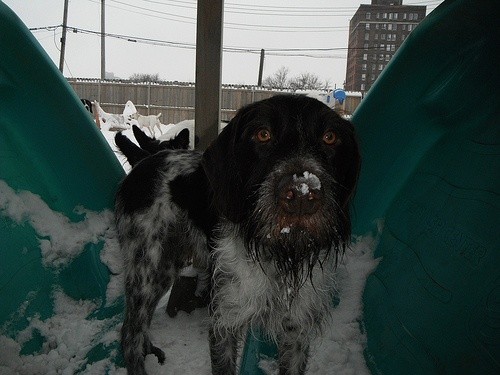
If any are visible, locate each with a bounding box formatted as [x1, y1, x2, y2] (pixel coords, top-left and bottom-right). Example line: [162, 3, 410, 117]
[80, 99, 93, 113]
[111, 93, 362, 375]
